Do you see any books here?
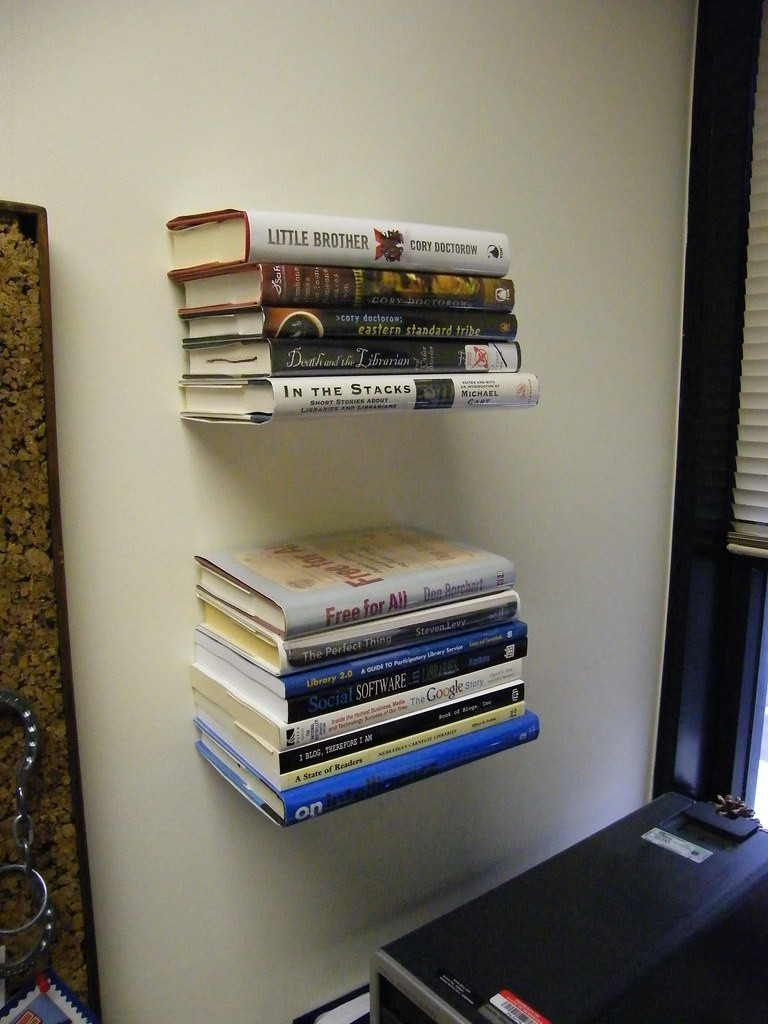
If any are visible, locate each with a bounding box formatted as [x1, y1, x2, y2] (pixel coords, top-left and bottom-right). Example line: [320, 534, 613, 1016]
[188, 524, 539, 829]
[165, 208, 540, 426]
